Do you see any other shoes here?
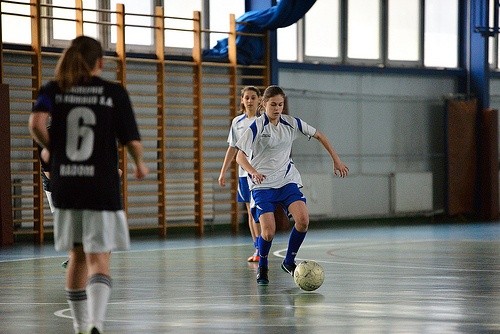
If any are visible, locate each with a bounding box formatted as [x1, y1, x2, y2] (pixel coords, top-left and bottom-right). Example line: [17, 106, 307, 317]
[247, 256, 255, 263]
[256, 263, 270, 286]
[252, 247, 261, 261]
[281, 260, 299, 278]
[88, 324, 104, 334]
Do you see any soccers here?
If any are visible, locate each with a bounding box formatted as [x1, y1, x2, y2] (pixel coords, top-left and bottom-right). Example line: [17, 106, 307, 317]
[292, 260, 325, 292]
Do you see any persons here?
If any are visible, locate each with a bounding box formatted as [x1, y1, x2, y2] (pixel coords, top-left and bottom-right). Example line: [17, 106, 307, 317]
[28, 35, 147, 334]
[218, 85, 351, 286]
